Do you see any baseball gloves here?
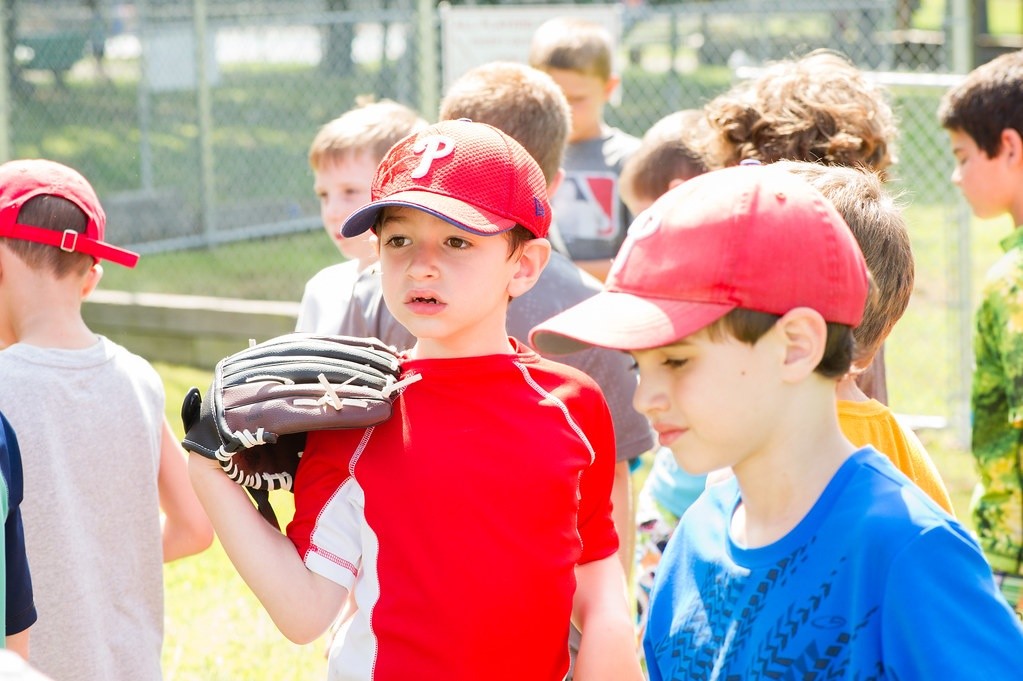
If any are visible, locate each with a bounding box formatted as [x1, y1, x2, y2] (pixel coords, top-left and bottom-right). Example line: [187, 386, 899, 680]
[174, 331, 423, 531]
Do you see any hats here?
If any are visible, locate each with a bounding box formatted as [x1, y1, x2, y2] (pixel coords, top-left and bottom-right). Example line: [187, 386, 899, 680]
[339, 118, 552, 238]
[0, 159, 140, 268]
[527, 158, 871, 355]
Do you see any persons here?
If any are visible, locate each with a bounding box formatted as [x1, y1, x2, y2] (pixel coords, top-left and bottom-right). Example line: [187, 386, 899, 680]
[185, 115, 646, 681]
[0, 156, 218, 680]
[0, 407, 40, 677]
[516, 158, 1023, 681]
[748, 158, 956, 523]
[516, 12, 898, 528]
[937, 38, 1023, 642]
[291, 94, 438, 336]
[438, 57, 657, 681]
